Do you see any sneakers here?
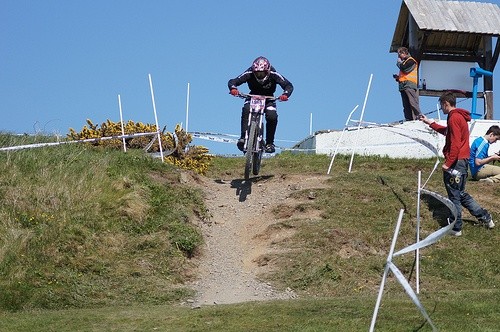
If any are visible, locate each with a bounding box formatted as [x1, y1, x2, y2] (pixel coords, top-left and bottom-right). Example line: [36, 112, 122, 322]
[449, 230, 463, 236]
[478, 219, 495, 228]
[237, 139, 245, 152]
[265, 144, 275, 153]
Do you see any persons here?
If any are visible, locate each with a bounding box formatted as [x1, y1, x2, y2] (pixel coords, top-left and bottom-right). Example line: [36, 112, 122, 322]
[392, 47, 422, 121]
[227, 56, 294, 153]
[418, 92, 495, 237]
[469, 125, 500, 183]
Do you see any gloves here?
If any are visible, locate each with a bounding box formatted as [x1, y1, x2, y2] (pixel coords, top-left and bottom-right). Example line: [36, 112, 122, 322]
[397, 57, 401, 63]
[231, 89, 239, 95]
[280, 94, 287, 101]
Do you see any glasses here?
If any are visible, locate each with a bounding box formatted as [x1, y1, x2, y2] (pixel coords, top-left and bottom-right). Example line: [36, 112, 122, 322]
[398, 53, 402, 57]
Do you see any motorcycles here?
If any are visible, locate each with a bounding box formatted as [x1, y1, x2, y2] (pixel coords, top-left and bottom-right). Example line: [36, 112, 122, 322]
[229, 91, 289, 182]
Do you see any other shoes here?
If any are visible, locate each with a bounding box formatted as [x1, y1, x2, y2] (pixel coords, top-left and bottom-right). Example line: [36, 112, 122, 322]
[479, 178, 494, 184]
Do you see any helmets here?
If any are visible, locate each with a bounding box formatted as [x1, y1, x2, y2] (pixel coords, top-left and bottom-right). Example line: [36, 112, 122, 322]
[252, 56, 270, 85]
